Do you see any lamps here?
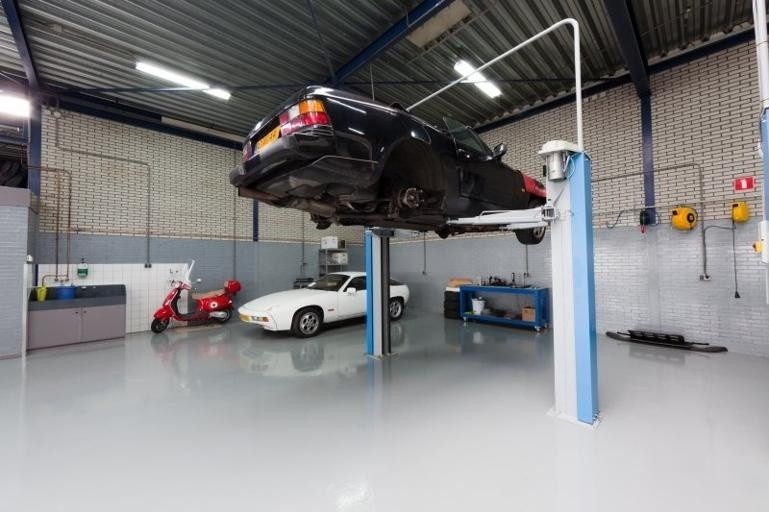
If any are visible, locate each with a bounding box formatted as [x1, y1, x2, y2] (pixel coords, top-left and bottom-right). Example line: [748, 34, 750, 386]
[454, 59, 502, 99]
[134, 61, 231, 101]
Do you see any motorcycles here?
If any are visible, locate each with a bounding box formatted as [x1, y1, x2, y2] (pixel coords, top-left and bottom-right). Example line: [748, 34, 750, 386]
[152, 270, 241, 334]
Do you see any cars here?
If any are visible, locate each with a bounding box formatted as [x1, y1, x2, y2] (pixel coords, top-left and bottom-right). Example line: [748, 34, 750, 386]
[237, 271, 410, 338]
[230, 86, 547, 243]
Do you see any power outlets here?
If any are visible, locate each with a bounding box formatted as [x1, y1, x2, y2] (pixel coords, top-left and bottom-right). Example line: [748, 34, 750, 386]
[523, 272, 529, 277]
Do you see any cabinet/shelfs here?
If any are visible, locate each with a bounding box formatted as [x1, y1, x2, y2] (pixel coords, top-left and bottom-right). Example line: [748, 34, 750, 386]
[444, 291, 461, 318]
[319, 248, 345, 278]
[459, 284, 550, 332]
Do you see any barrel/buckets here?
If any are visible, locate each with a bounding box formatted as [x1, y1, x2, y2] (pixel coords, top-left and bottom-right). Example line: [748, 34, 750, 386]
[77, 258, 88, 277]
[55, 286, 76, 300]
[472, 298, 487, 315]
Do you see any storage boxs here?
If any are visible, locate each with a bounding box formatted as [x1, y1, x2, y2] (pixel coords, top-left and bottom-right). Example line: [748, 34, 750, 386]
[522, 306, 536, 322]
[321, 236, 342, 249]
[331, 252, 348, 264]
